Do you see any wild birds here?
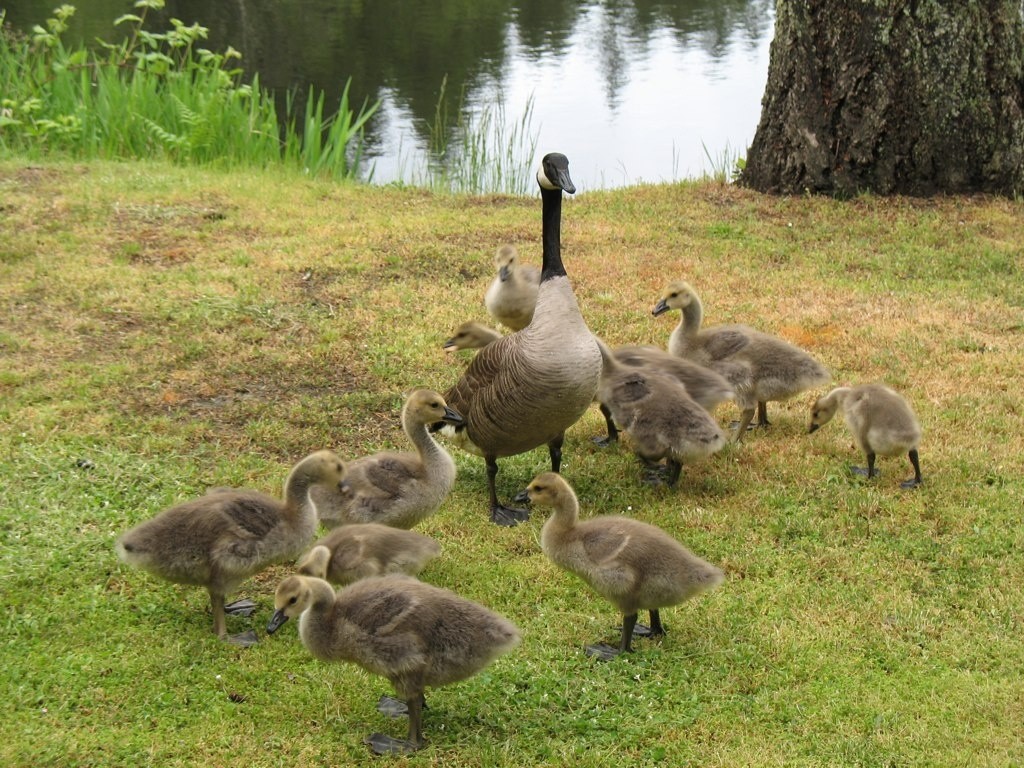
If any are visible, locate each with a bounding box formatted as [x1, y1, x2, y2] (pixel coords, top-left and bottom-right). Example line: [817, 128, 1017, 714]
[307, 386, 465, 534]
[806, 380, 923, 492]
[512, 469, 726, 666]
[120, 455, 349, 643]
[292, 520, 446, 589]
[649, 277, 836, 447]
[441, 319, 506, 355]
[424, 150, 604, 530]
[483, 243, 543, 334]
[265, 572, 526, 759]
[592, 331, 725, 491]
[591, 344, 737, 450]
